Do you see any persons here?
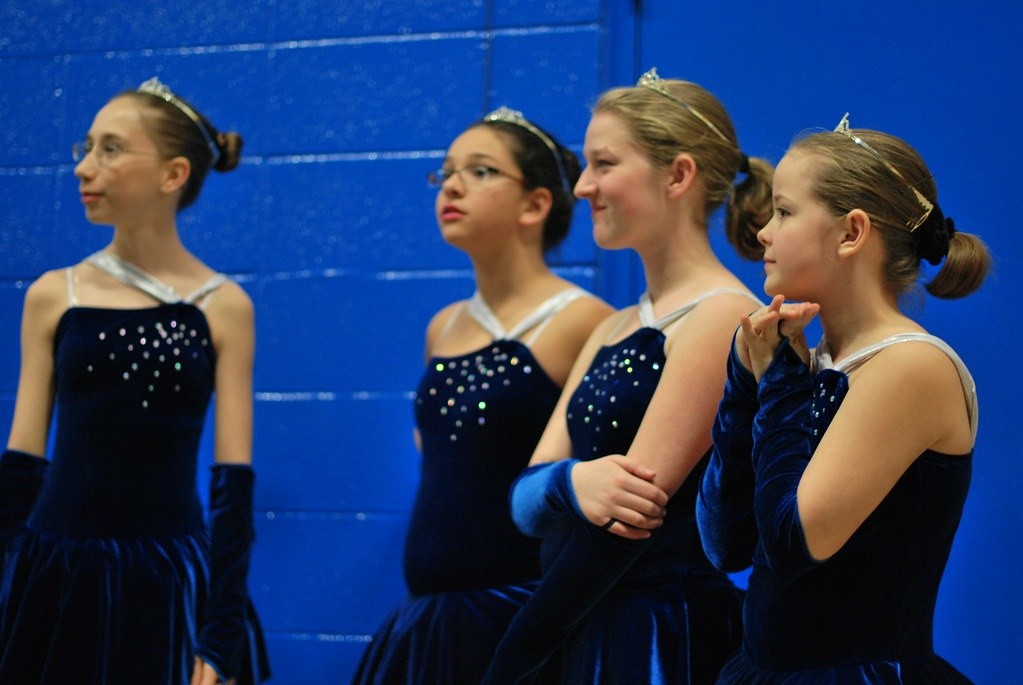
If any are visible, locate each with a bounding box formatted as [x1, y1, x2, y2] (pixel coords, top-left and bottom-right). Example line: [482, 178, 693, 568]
[484, 67, 776, 685]
[694, 115, 988, 685]
[347, 105, 617, 685]
[0, 74, 274, 685]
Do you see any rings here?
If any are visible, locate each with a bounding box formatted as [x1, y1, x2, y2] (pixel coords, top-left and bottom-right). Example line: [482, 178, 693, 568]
[602, 518, 614, 531]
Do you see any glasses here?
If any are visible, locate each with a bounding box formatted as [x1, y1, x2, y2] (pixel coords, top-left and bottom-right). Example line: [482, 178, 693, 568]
[423, 164, 536, 192]
[71, 140, 179, 167]
